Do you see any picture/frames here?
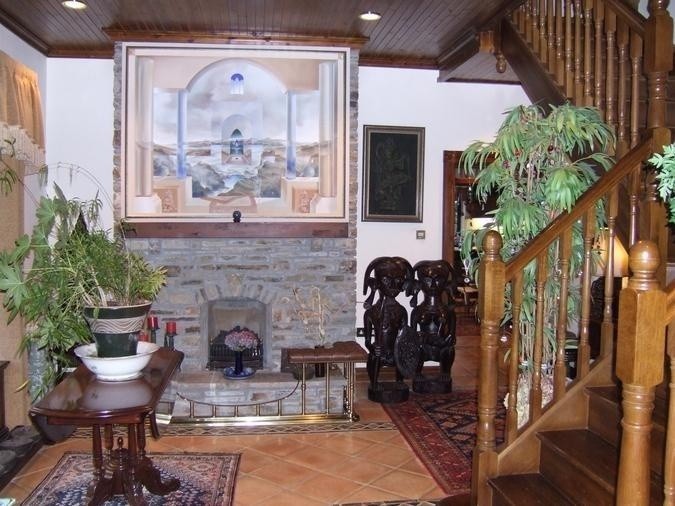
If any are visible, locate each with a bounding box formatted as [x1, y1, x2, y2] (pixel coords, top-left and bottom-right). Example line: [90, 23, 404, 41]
[361, 124, 426, 223]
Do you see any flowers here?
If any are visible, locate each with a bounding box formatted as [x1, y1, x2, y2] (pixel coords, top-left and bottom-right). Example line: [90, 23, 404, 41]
[224, 331, 259, 352]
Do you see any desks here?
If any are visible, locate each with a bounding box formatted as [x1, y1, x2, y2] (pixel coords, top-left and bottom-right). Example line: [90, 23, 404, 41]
[29, 347, 185, 506]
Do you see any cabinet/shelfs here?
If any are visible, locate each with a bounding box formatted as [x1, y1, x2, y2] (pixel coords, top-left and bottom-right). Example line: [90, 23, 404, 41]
[443, 150, 545, 337]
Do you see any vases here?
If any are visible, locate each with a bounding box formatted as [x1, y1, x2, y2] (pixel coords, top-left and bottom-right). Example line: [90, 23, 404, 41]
[223, 349, 256, 379]
[314, 345, 326, 377]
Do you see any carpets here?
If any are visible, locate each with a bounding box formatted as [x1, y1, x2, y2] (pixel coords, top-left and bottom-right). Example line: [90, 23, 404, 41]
[19, 449, 241, 506]
[380, 383, 511, 496]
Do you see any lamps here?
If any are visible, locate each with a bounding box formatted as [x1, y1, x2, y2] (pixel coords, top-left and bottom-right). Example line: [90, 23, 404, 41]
[589, 225, 629, 278]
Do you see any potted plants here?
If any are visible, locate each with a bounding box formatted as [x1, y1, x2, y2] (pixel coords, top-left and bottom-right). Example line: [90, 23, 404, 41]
[13, 361, 78, 444]
[1, 137, 169, 357]
